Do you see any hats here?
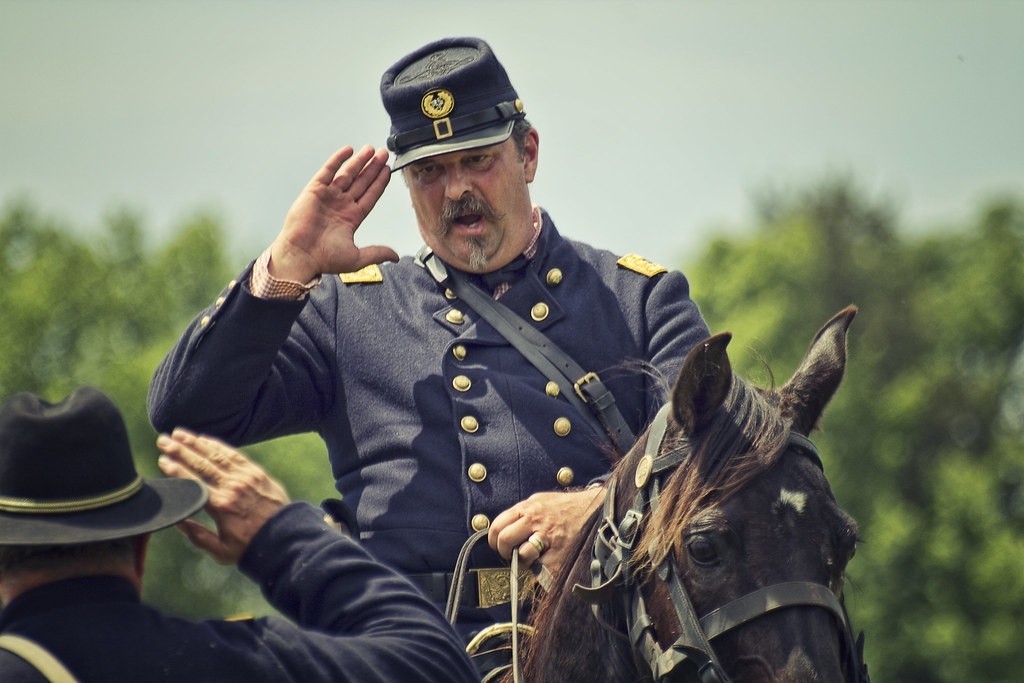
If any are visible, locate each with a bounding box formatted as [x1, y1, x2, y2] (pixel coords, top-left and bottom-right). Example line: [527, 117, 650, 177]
[0, 387, 210, 546]
[379, 38, 524, 172]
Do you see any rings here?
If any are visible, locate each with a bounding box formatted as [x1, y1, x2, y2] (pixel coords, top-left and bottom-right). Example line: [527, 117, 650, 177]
[527, 533, 545, 553]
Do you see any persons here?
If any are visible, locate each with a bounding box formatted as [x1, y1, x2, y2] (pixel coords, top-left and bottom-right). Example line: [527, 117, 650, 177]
[146, 36, 733, 682]
[0, 387, 479, 682]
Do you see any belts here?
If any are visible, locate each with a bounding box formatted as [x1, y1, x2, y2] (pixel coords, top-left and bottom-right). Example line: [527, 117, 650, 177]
[404, 568, 547, 610]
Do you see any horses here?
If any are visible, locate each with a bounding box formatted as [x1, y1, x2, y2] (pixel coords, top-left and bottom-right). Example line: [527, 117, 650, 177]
[490, 303, 871, 683]
